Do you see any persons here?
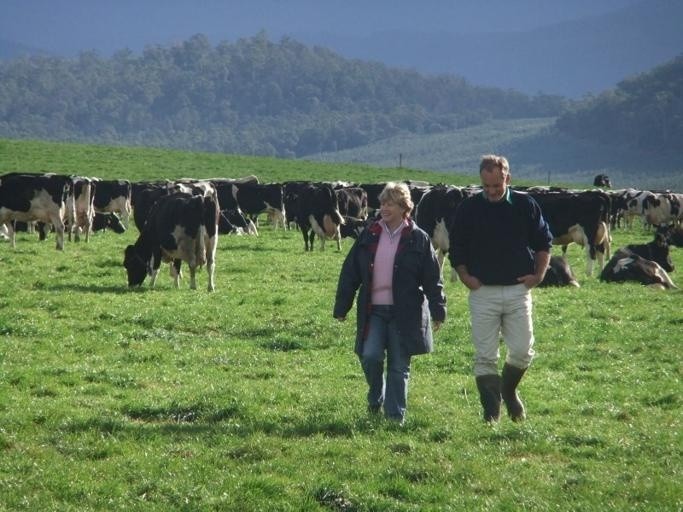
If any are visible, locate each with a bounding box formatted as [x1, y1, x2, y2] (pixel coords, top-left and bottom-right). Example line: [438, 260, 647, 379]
[329, 179, 448, 428]
[447, 150, 555, 424]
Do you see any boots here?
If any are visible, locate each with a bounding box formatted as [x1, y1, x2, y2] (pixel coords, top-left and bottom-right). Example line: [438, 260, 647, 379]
[502, 362, 529, 424]
[476, 374, 501, 425]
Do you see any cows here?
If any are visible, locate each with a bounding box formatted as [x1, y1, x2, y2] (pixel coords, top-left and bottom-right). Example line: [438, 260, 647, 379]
[0, 172, 683, 255]
[122, 191, 219, 293]
[599, 231, 683, 295]
[522, 189, 611, 282]
[530, 251, 580, 289]
[414, 182, 482, 285]
